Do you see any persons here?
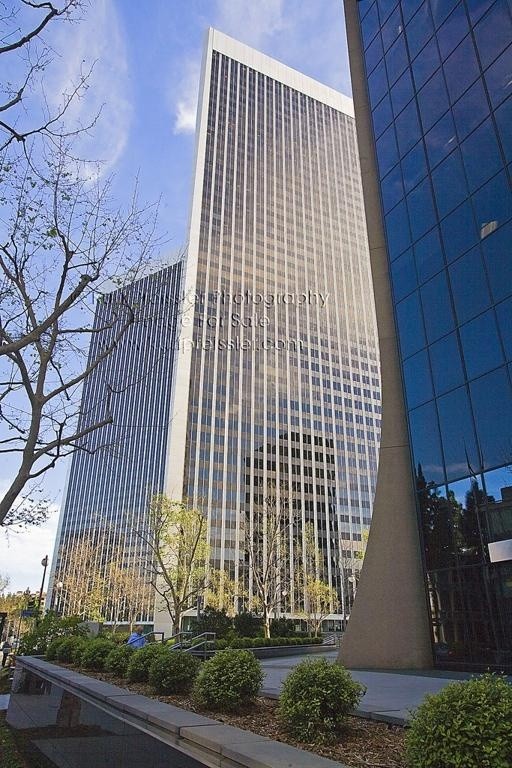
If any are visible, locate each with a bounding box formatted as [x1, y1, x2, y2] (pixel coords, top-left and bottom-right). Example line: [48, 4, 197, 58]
[2, 640, 12, 667]
[11, 643, 16, 648]
[127, 626, 146, 650]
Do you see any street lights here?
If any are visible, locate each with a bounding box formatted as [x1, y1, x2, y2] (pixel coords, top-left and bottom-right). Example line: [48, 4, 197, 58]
[35, 553, 50, 612]
[56, 578, 64, 613]
